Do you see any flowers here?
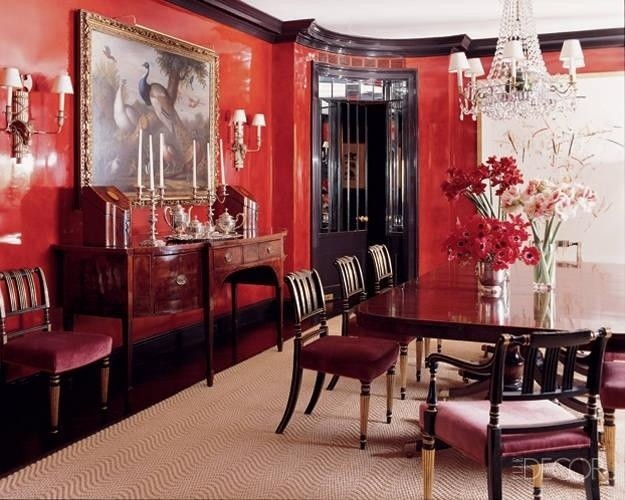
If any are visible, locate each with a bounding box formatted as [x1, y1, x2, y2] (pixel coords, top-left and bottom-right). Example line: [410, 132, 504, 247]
[437, 151, 600, 268]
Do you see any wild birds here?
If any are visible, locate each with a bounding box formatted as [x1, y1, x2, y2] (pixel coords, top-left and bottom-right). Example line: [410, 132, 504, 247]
[102, 44, 117, 64]
[112, 78, 139, 139]
[138, 61, 193, 138]
[189, 97, 206, 108]
[163, 133, 178, 175]
[184, 128, 203, 185]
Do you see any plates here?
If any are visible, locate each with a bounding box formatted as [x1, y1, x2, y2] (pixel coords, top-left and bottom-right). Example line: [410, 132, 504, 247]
[217, 234, 243, 239]
[165, 234, 192, 240]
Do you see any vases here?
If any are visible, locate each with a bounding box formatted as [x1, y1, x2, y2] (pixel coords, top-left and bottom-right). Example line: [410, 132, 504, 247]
[529, 243, 560, 330]
[477, 263, 507, 297]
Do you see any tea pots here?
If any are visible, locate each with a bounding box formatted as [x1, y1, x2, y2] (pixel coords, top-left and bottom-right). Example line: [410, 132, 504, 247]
[191, 215, 216, 240]
[164, 201, 193, 237]
[216, 208, 245, 235]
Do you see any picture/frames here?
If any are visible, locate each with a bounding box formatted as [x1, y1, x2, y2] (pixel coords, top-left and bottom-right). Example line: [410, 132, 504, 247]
[73, 6, 222, 210]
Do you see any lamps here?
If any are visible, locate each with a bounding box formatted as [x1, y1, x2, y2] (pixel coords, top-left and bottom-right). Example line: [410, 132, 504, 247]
[225, 104, 270, 172]
[447, 0, 589, 123]
[0, 63, 77, 164]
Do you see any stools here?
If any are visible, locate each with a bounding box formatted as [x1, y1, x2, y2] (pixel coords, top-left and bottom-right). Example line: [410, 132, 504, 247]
[602, 351, 625, 486]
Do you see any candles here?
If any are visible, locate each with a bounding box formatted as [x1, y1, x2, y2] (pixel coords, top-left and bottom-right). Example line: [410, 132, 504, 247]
[191, 137, 225, 191]
[133, 128, 165, 190]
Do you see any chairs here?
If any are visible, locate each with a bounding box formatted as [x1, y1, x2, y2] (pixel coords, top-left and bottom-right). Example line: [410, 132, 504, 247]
[276, 240, 444, 452]
[0, 265, 117, 438]
[415, 324, 612, 499]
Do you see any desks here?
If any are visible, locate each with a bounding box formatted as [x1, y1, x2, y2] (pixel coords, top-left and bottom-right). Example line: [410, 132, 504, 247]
[59, 225, 293, 406]
[353, 257, 625, 459]
